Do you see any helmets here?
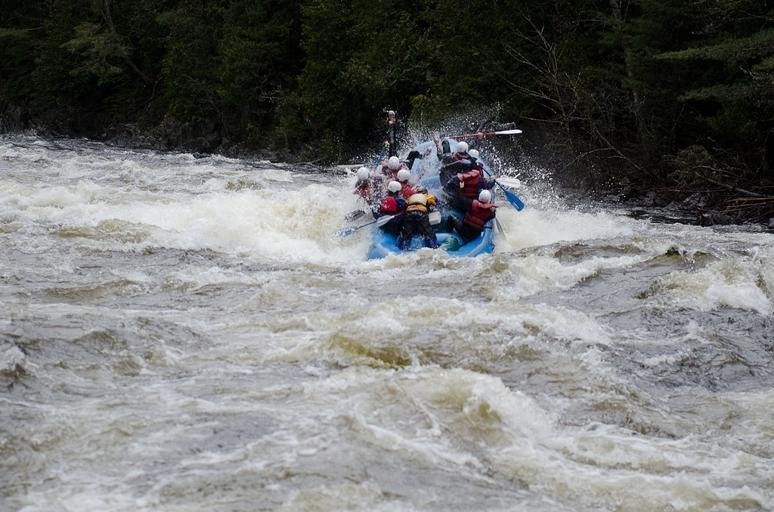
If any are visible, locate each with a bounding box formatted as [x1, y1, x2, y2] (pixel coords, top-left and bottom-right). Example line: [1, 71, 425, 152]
[388, 156, 400, 170]
[357, 167, 370, 181]
[468, 149, 479, 160]
[416, 186, 428, 196]
[397, 169, 410, 182]
[479, 189, 492, 204]
[387, 181, 402, 194]
[457, 141, 468, 153]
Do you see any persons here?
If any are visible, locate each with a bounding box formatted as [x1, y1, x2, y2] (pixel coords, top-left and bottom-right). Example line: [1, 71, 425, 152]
[353, 133, 497, 248]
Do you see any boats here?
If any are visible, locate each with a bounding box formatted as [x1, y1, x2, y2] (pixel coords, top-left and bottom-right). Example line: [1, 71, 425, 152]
[364, 138, 495, 264]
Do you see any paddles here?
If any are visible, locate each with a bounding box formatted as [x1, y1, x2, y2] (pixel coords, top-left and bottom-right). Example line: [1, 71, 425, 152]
[377, 212, 403, 227]
[332, 219, 377, 238]
[336, 164, 377, 169]
[495, 215, 506, 239]
[464, 150, 524, 211]
[450, 129, 522, 138]
